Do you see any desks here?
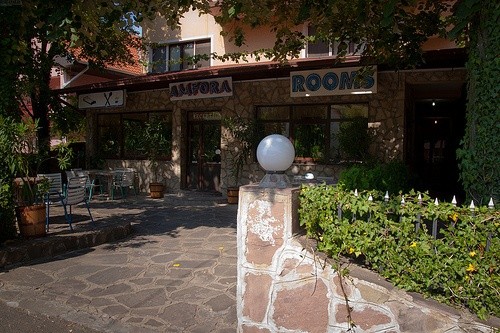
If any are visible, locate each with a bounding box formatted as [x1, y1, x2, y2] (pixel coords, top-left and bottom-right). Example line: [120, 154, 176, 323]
[37, 191, 73, 232]
[87, 169, 131, 201]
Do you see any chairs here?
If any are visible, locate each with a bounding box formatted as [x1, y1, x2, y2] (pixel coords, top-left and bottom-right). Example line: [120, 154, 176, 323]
[76, 170, 104, 201]
[65, 170, 87, 201]
[52, 174, 95, 228]
[70, 168, 83, 177]
[36, 172, 67, 222]
[111, 169, 139, 201]
[107, 166, 128, 198]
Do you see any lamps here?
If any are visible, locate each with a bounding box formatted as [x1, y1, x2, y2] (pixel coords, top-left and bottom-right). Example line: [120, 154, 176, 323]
[256, 133, 296, 188]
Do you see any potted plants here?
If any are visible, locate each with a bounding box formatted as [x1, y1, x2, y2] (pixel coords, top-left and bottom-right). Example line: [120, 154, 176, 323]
[145, 148, 175, 199]
[221, 141, 253, 205]
